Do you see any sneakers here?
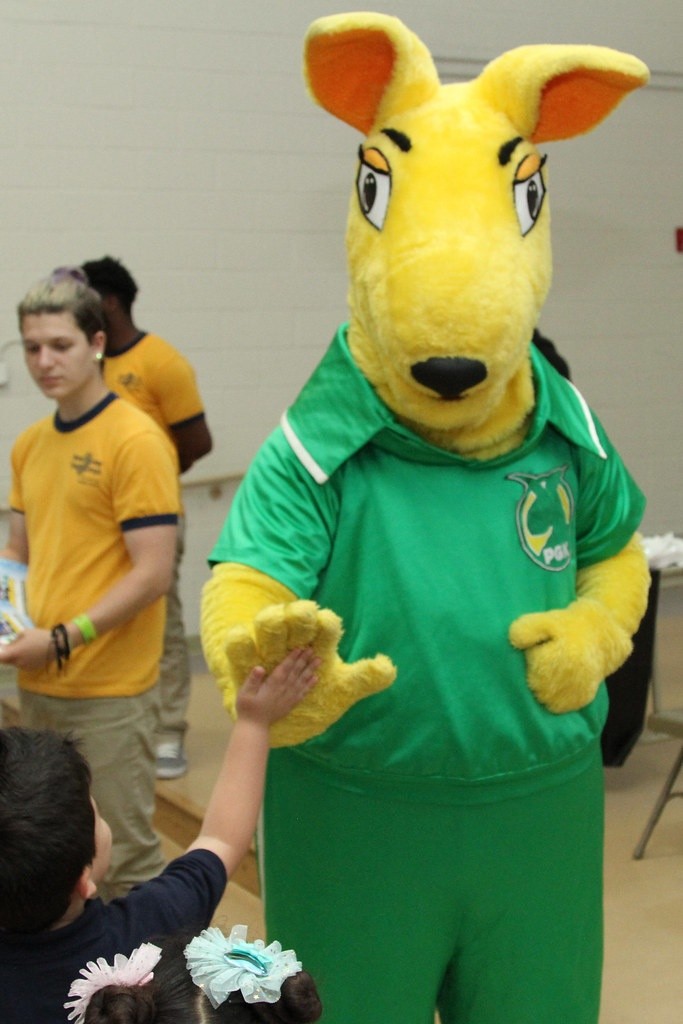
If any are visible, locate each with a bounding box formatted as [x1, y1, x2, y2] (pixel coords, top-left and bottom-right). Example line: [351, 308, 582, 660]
[154, 738, 185, 781]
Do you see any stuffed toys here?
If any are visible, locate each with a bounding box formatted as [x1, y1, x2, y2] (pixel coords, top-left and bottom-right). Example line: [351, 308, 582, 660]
[198, 12, 653, 1024]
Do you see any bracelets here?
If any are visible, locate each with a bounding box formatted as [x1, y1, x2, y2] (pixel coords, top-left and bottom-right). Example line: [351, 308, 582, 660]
[73, 612, 97, 646]
[52, 622, 70, 669]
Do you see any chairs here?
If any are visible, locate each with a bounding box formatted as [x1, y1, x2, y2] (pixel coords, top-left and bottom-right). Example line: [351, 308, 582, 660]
[631, 567, 683, 860]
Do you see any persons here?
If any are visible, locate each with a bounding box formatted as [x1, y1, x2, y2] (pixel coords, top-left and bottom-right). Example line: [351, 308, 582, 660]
[73, 258, 214, 778]
[0, 274, 186, 926]
[68, 927, 322, 1024]
[0, 646, 323, 1024]
